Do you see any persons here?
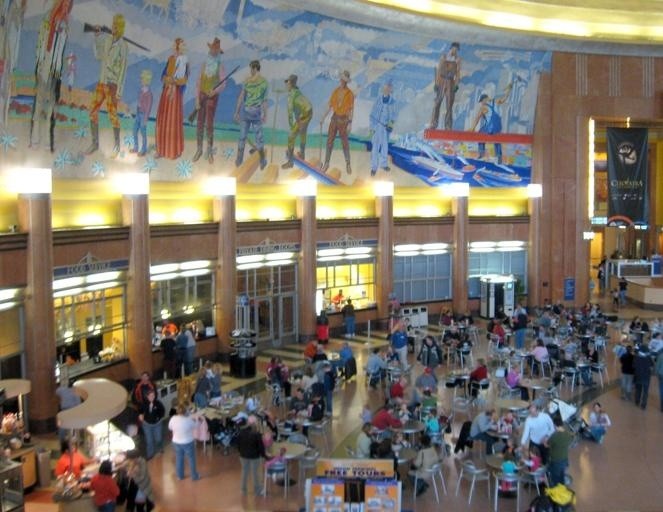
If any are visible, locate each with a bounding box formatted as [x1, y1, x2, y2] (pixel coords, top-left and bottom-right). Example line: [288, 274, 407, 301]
[469, 79, 512, 164]
[86, 14, 129, 159]
[0, 0, 27, 139]
[319, 71, 353, 175]
[155, 38, 190, 160]
[129, 70, 153, 156]
[368, 85, 399, 177]
[0, 288, 661, 511]
[29, 1, 74, 152]
[193, 37, 226, 164]
[281, 75, 313, 169]
[428, 42, 461, 130]
[233, 61, 270, 171]
